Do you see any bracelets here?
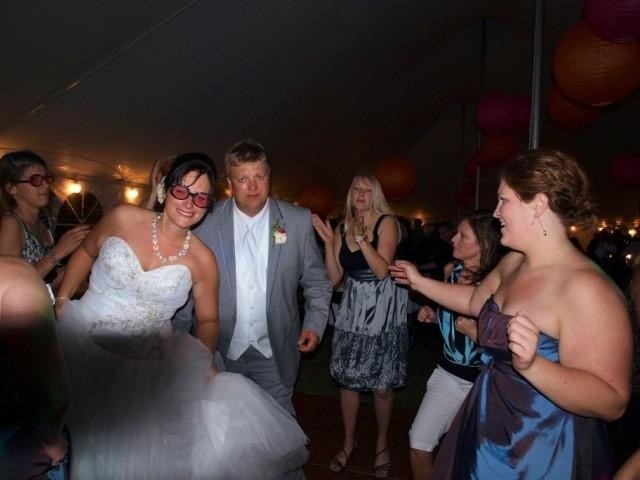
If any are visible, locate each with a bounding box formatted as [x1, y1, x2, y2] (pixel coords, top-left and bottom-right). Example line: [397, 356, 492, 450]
[46, 248, 61, 267]
[353, 234, 367, 243]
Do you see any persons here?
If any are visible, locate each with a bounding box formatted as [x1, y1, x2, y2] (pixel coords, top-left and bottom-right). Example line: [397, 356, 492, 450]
[610, 446, 639, 480]
[148, 156, 164, 212]
[387, 142, 632, 480]
[0, 253, 70, 479]
[307, 166, 410, 479]
[315, 208, 640, 328]
[54, 153, 308, 480]
[407, 208, 508, 480]
[0, 144, 95, 283]
[191, 136, 334, 418]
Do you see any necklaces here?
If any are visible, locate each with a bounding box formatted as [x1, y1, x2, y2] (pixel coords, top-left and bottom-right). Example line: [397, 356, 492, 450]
[150, 211, 193, 264]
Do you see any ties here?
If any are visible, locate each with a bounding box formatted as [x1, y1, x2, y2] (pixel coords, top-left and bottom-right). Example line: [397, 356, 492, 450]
[243, 222, 258, 260]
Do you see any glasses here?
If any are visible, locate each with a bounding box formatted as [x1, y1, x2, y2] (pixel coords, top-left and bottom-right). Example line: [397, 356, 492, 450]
[18, 172, 56, 186]
[169, 184, 216, 208]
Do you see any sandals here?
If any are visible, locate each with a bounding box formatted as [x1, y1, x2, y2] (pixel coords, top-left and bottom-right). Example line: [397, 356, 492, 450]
[330, 440, 358, 473]
[374, 442, 391, 476]
